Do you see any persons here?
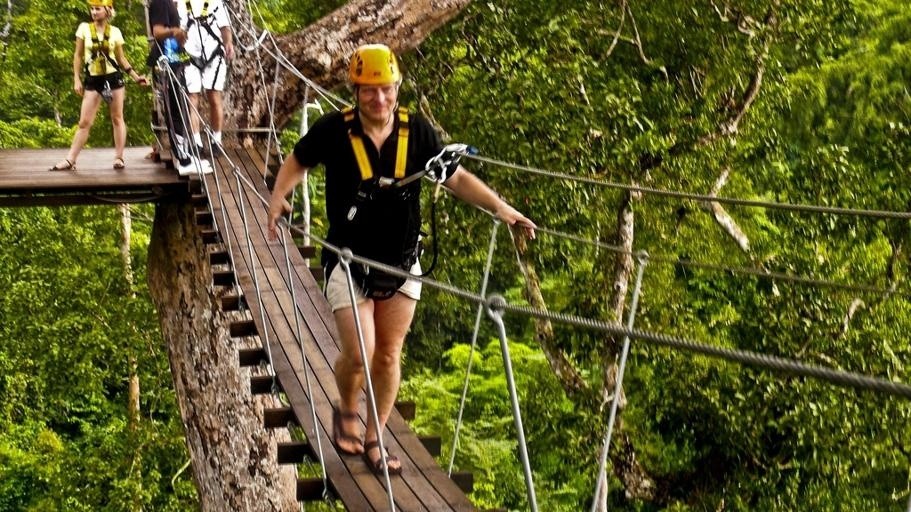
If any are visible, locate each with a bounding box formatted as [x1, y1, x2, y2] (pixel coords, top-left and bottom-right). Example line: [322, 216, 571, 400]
[45, 0, 151, 170]
[146, 0, 215, 178]
[178, 1, 236, 158]
[262, 35, 541, 475]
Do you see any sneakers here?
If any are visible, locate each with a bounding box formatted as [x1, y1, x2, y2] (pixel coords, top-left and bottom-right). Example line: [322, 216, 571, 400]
[178, 162, 214, 178]
[191, 143, 205, 159]
[211, 141, 224, 159]
[172, 154, 211, 170]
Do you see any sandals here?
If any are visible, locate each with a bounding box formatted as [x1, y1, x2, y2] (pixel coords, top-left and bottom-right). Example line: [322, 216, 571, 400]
[363, 438, 405, 475]
[49, 159, 79, 172]
[331, 398, 367, 457]
[112, 157, 126, 170]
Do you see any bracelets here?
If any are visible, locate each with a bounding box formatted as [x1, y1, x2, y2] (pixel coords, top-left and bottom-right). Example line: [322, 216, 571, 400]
[125, 67, 133, 74]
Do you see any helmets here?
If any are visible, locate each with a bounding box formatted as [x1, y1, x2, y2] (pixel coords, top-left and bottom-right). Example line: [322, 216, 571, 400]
[348, 43, 404, 87]
[86, 0, 114, 7]
